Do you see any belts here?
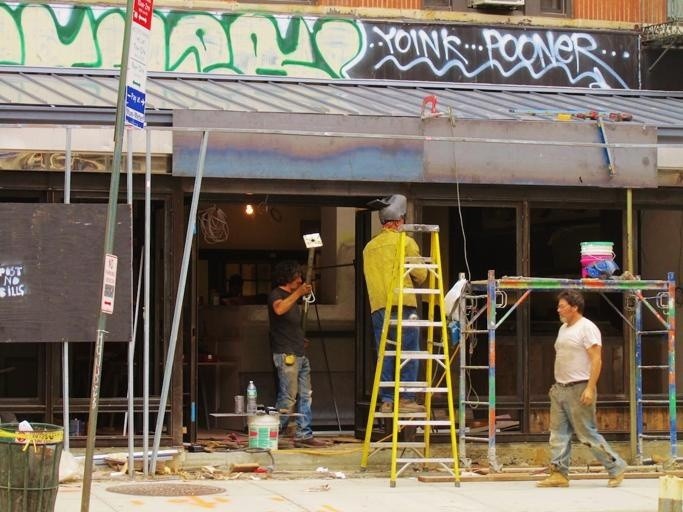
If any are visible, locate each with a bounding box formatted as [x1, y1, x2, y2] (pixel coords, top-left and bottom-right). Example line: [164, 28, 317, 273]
[555, 379, 589, 388]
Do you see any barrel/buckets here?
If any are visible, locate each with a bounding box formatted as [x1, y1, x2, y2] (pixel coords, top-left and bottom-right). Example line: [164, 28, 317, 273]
[0, 422, 66, 512]
[657, 475, 683, 511]
[580, 241, 614, 277]
[246, 414, 279, 450]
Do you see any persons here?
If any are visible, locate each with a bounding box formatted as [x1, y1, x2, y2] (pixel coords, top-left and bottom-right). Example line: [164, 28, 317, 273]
[268, 262, 327, 449]
[363, 216, 428, 413]
[536, 289, 628, 488]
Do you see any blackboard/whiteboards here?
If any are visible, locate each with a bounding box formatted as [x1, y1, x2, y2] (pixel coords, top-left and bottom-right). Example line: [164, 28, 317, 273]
[0, 203, 134, 343]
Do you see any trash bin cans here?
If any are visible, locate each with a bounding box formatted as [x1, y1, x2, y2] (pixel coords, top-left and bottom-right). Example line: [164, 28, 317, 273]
[0, 423, 65, 512]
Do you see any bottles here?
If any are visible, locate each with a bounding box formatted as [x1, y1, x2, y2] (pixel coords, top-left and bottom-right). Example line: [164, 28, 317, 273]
[247, 381, 257, 414]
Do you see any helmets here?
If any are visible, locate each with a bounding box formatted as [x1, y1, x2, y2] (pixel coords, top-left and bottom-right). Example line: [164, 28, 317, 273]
[368, 194, 408, 220]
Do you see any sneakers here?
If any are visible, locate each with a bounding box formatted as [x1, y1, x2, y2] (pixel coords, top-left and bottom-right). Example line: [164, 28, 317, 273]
[535, 471, 571, 488]
[379, 401, 394, 414]
[606, 461, 629, 488]
[293, 434, 327, 448]
[397, 396, 427, 414]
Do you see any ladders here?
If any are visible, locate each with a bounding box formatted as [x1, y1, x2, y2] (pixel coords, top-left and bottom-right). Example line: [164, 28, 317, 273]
[360, 224, 461, 488]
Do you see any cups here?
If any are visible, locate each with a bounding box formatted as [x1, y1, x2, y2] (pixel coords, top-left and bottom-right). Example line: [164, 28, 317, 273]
[234, 396, 244, 414]
